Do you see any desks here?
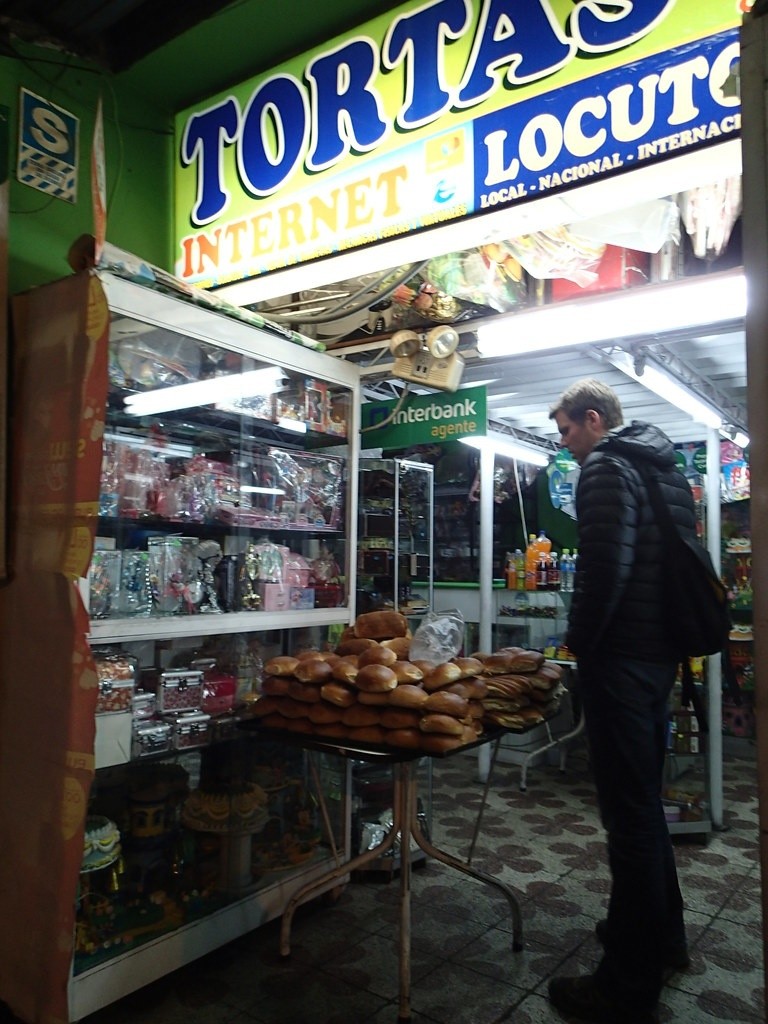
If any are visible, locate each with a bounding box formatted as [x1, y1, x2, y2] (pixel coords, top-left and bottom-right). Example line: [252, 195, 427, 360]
[521, 658, 587, 791]
[277, 737, 524, 1024]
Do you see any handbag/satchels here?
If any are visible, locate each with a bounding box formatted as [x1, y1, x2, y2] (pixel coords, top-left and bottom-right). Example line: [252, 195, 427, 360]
[663, 536, 735, 659]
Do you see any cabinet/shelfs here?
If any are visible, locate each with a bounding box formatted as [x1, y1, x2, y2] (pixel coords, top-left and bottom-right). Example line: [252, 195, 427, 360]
[94, 386, 347, 541]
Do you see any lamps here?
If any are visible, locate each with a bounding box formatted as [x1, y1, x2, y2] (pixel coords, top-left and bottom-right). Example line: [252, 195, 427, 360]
[390, 324, 466, 393]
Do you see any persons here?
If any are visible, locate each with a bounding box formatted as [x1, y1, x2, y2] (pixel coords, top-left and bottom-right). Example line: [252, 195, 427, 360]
[547, 377, 702, 1021]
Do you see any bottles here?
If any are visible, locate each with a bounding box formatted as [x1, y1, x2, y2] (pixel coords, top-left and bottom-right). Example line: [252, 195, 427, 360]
[504, 531, 579, 592]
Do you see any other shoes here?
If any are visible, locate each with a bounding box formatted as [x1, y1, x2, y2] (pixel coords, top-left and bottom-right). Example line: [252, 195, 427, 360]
[549, 975, 658, 1024]
[594, 920, 690, 973]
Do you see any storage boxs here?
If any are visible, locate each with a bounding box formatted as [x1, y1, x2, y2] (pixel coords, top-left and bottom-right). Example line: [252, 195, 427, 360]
[94, 669, 209, 768]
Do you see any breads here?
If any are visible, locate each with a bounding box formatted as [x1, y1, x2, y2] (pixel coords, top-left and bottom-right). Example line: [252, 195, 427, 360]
[252, 609, 565, 754]
